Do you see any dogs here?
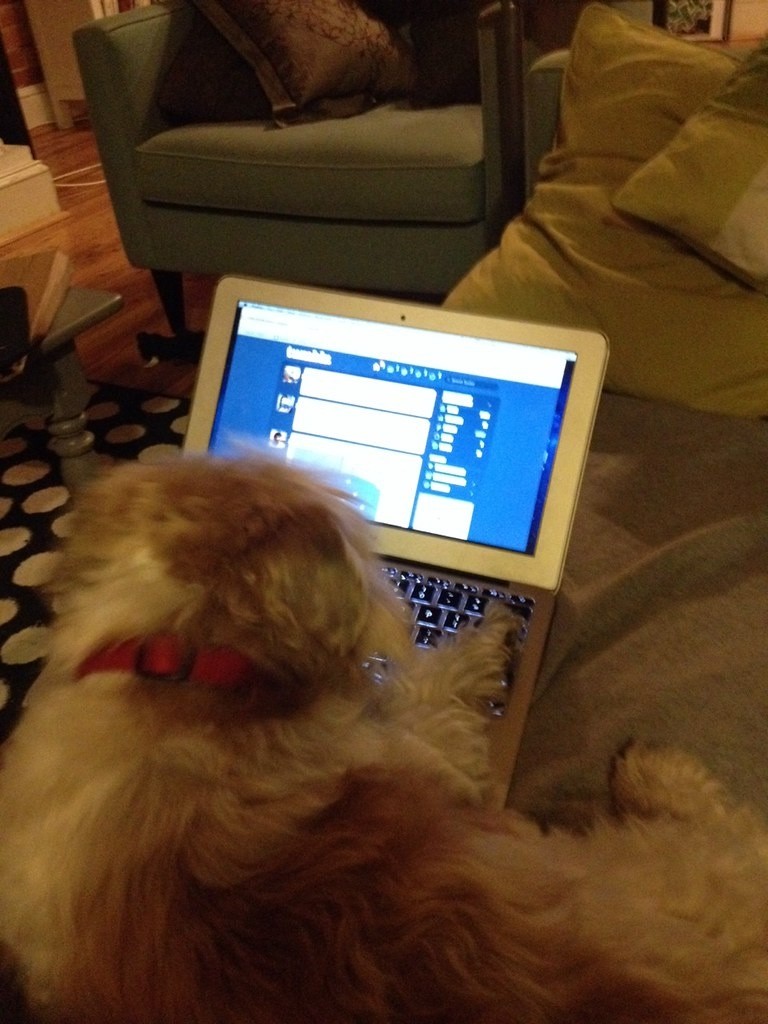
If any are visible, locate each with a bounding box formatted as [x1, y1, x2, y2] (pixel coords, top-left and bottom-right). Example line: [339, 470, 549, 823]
[1, 445, 768, 1023]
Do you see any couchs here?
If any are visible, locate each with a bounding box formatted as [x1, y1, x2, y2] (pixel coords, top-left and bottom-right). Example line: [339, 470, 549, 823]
[438, 38, 768, 896]
[71, 0, 520, 336]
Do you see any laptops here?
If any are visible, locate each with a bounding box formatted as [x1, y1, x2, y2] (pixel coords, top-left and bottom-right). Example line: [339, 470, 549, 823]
[177, 273, 610, 812]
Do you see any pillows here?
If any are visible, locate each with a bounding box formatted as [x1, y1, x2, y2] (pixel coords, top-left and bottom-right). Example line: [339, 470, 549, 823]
[433, 2, 768, 422]
[196, 0, 417, 127]
[612, 40, 768, 292]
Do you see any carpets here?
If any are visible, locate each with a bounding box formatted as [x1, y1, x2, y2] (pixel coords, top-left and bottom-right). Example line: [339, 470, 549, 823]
[0, 378, 204, 744]
